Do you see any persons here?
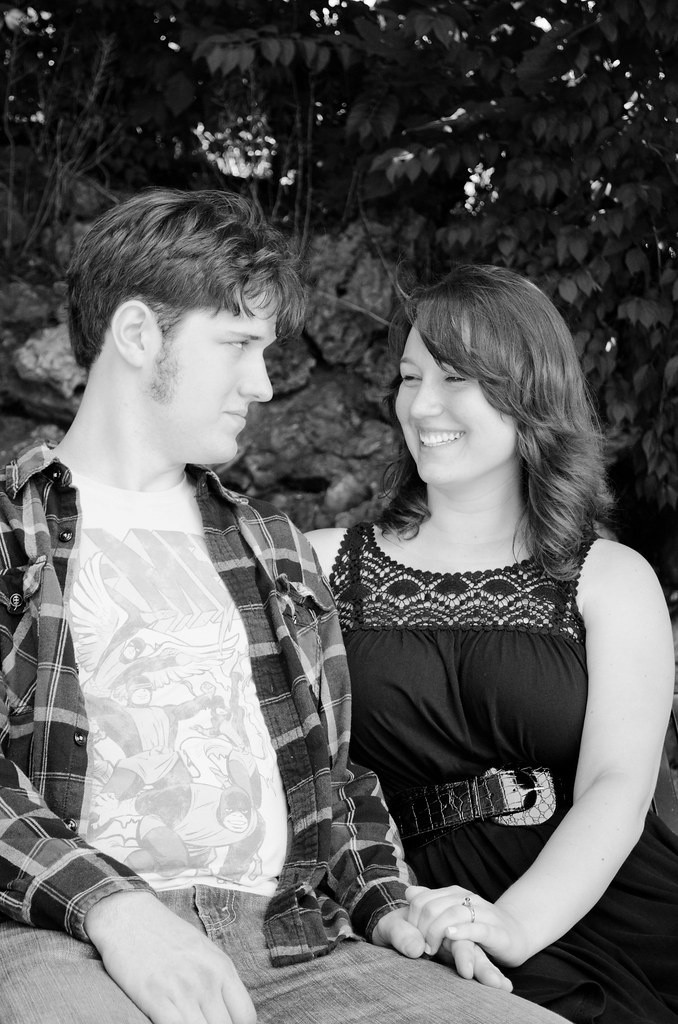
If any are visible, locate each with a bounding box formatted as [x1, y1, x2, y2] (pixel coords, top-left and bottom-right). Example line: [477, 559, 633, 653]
[301, 263, 678, 1024]
[1, 188, 579, 1024]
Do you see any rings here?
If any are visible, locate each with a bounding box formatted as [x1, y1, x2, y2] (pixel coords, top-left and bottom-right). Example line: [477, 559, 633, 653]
[462, 897, 475, 922]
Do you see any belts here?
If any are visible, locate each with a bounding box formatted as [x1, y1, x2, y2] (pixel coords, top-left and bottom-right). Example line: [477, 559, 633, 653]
[391, 764, 557, 852]
